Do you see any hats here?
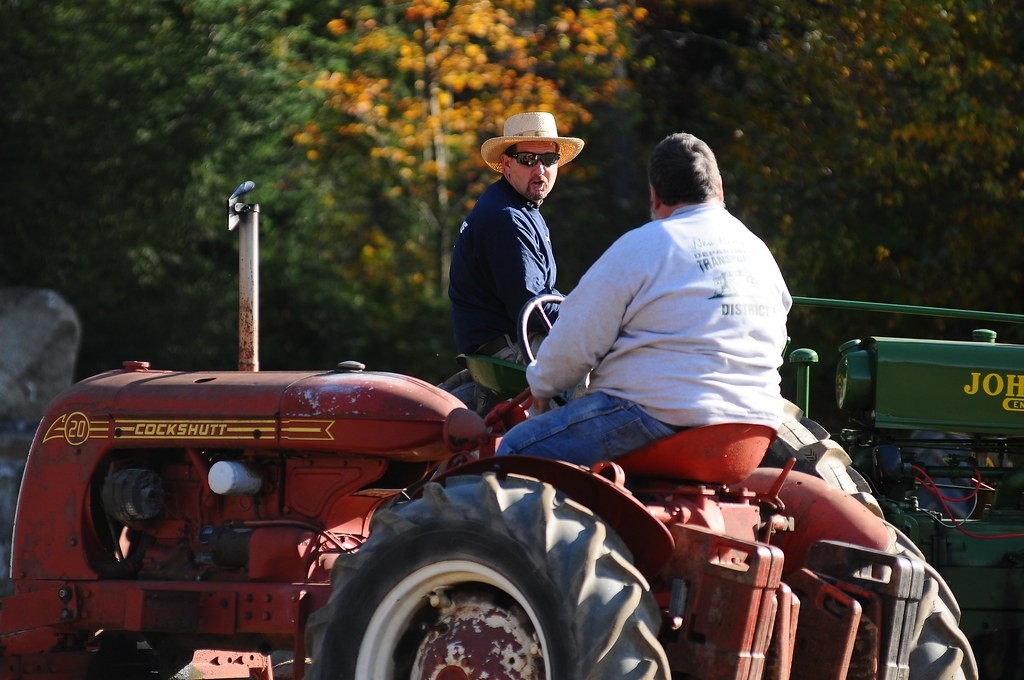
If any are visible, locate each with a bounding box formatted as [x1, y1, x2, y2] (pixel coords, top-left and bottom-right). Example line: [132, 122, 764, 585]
[479, 112, 585, 173]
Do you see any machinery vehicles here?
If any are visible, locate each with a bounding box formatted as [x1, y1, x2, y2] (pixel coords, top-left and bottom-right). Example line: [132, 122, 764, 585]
[1, 291, 1024, 676]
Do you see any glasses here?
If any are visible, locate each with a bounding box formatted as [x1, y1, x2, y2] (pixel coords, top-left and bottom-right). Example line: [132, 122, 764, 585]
[505, 151, 560, 166]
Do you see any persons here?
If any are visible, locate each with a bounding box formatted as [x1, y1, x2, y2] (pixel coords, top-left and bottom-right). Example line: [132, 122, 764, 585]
[448, 112, 589, 402]
[496, 131, 795, 466]
